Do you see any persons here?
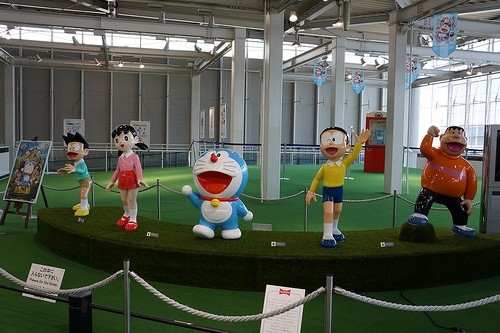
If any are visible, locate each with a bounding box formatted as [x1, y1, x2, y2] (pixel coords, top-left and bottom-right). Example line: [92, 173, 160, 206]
[57, 131, 93, 217]
[407, 125, 479, 240]
[304, 126, 372, 248]
[104, 124, 149, 232]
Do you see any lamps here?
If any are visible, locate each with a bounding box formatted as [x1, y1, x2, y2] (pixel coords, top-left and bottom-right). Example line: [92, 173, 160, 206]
[118, 58, 124, 67]
[375, 59, 381, 68]
[94, 58, 101, 66]
[140, 60, 145, 68]
[289, 4, 298, 22]
[361, 57, 367, 67]
[35, 54, 42, 63]
[476, 68, 483, 76]
[348, 75, 352, 79]
[466, 64, 473, 76]
[332, 20, 344, 27]
